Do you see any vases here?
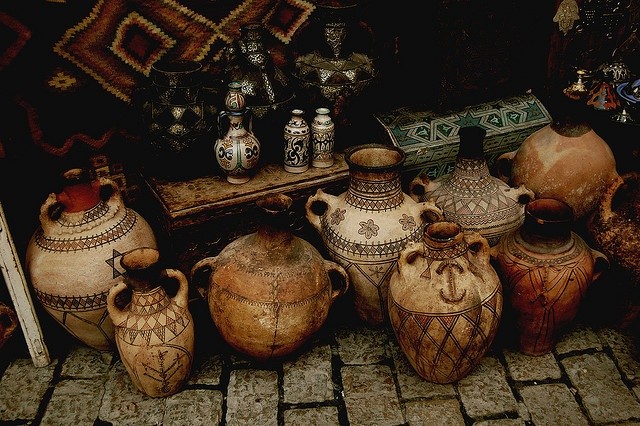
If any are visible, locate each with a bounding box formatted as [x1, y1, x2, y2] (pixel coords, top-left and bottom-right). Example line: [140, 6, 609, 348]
[187, 191, 348, 369]
[221, 21, 301, 131]
[588, 151, 639, 329]
[109, 248, 192, 397]
[25, 167, 158, 352]
[562, 70, 588, 115]
[488, 199, 610, 355]
[409, 125, 534, 263]
[132, 59, 223, 162]
[304, 144, 444, 326]
[290, 2, 380, 133]
[606, 78, 640, 168]
[584, 69, 621, 135]
[497, 87, 618, 230]
[387, 222, 503, 384]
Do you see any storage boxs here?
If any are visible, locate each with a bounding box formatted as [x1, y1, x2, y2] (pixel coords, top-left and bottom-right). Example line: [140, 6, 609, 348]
[372, 92, 552, 194]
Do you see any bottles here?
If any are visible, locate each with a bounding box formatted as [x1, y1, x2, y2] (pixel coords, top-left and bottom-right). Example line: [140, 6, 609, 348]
[313, 108, 334, 169]
[285, 109, 310, 174]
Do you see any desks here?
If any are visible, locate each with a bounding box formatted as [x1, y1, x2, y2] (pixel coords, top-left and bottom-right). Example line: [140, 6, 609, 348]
[137, 151, 351, 308]
